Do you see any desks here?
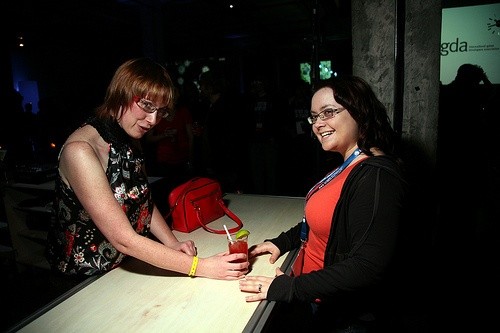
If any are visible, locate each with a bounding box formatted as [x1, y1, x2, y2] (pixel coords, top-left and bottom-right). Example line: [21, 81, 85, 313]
[4, 192, 306, 333]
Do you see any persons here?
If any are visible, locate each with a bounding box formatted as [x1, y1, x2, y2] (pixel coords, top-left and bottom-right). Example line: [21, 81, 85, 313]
[2, 64, 500, 177]
[240, 76, 410, 328]
[52, 58, 249, 282]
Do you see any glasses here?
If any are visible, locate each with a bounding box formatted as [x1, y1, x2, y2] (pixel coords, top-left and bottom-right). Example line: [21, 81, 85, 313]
[132, 95, 171, 119]
[307, 106, 345, 125]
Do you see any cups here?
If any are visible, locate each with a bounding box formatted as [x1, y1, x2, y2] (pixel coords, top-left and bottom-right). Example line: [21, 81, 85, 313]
[227, 234, 248, 275]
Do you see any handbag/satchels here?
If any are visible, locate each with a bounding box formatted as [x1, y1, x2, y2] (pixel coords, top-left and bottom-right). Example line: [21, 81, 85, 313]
[164, 178, 243, 234]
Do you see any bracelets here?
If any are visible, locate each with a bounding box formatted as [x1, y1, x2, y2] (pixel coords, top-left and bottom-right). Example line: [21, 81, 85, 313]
[189, 254, 200, 279]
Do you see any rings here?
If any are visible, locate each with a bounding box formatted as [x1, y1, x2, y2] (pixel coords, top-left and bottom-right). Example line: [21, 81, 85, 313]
[257, 283, 264, 293]
